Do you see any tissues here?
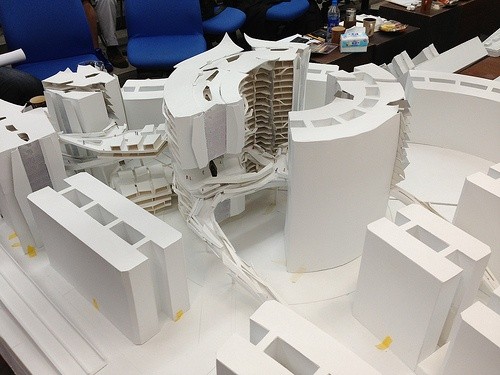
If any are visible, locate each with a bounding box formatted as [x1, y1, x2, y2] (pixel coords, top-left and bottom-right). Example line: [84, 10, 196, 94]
[339, 25, 370, 53]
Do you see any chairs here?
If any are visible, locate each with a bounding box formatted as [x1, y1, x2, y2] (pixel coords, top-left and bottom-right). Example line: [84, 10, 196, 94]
[0, 0, 341, 91]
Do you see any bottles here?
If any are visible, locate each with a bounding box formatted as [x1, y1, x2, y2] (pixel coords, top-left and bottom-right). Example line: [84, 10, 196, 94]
[327, 0, 340, 40]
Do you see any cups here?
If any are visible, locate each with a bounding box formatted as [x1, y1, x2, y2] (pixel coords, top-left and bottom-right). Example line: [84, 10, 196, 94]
[344, 8, 356, 29]
[363, 17, 376, 36]
[421, 0, 432, 13]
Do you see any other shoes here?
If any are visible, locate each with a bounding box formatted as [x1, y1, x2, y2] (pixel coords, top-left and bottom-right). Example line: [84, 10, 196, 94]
[107, 45, 129, 68]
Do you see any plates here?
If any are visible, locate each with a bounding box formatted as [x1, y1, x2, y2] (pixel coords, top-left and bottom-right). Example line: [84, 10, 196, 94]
[379, 25, 409, 33]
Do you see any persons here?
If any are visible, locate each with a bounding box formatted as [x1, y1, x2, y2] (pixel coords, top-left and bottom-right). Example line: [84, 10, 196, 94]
[95, 0, 130, 69]
[0, 66, 47, 108]
[81, 0, 113, 73]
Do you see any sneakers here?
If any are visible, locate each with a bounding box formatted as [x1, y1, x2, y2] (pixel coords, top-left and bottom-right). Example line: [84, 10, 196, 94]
[95, 48, 112, 70]
